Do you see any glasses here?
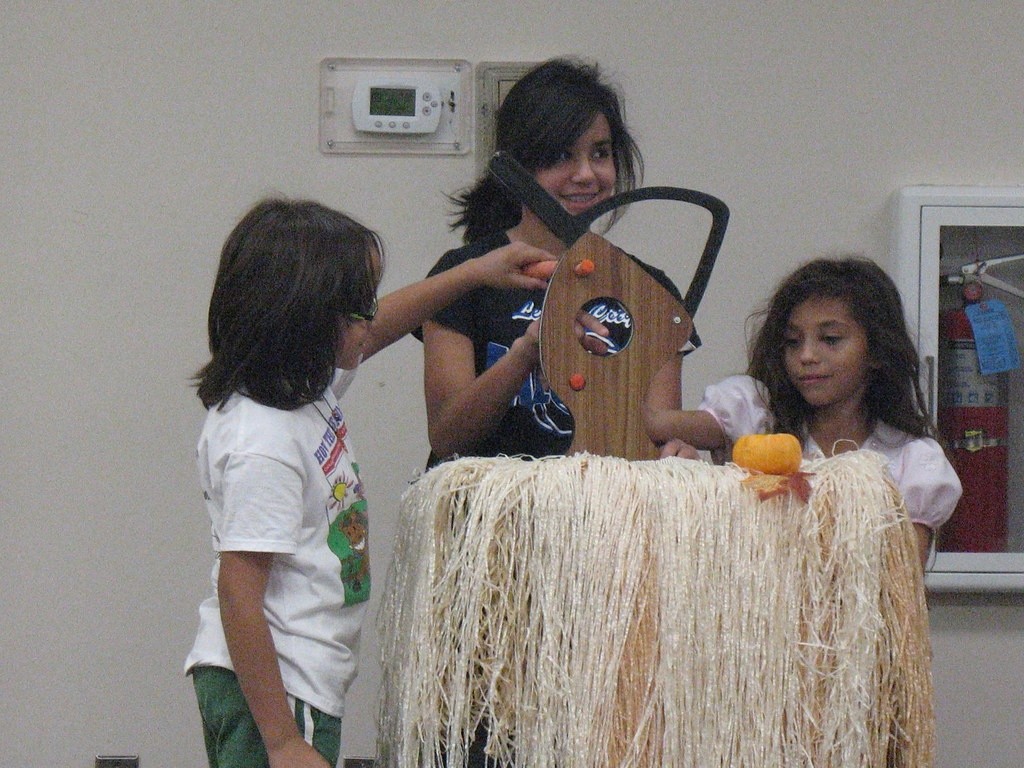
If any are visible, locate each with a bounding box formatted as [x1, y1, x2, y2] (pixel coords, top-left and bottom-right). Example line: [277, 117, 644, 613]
[329, 295, 379, 323]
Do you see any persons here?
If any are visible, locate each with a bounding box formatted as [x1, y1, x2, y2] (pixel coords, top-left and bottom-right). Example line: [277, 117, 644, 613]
[408, 55, 964, 768]
[182, 198, 556, 768]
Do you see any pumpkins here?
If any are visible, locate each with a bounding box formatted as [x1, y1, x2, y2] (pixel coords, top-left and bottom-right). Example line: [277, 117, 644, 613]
[732, 421, 803, 475]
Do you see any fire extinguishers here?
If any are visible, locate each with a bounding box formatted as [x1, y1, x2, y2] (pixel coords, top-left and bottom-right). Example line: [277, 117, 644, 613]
[937, 252, 1024, 553]
